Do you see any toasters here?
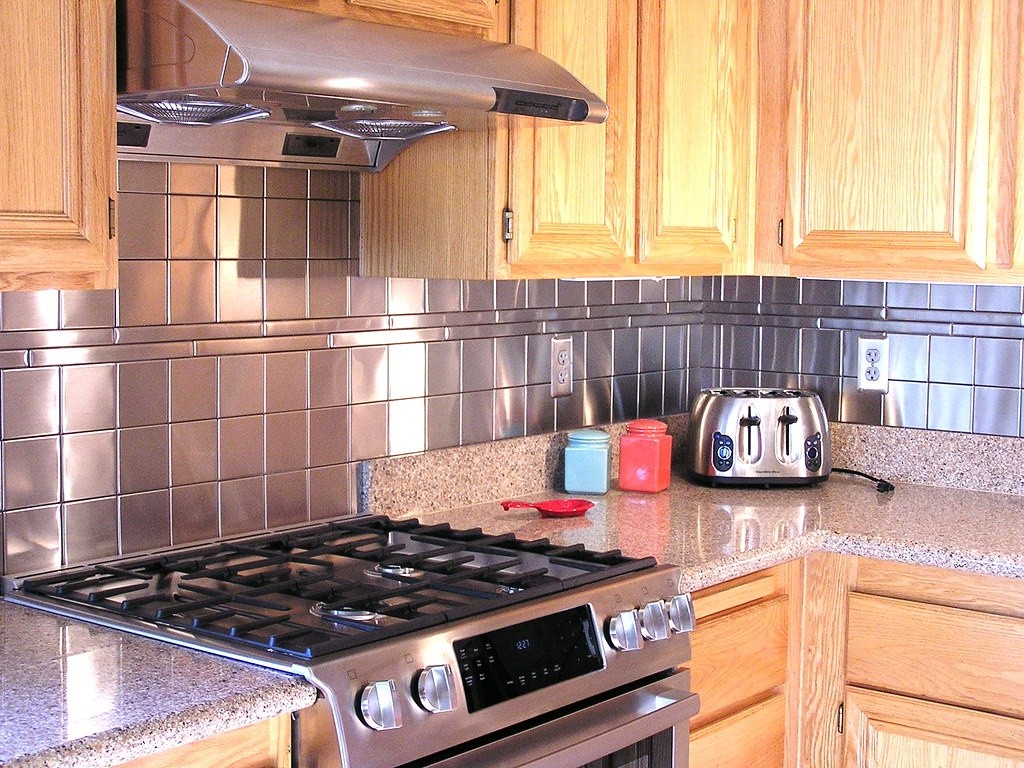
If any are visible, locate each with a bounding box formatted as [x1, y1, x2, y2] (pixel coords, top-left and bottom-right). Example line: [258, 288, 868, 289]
[685, 387, 896, 494]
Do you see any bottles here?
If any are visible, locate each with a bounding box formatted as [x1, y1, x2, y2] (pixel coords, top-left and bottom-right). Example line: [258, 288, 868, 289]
[618, 418, 673, 495]
[564, 430, 610, 496]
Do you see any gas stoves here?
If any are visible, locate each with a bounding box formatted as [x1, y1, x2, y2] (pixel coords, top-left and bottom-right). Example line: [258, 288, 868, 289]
[21, 515, 692, 768]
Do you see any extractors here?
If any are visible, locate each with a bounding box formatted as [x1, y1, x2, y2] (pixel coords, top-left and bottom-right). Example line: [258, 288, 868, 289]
[116, 0, 609, 172]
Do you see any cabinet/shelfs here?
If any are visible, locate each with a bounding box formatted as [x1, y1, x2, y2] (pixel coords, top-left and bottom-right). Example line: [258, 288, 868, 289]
[111, 712, 292, 768]
[242, 0, 1024, 288]
[674, 549, 1024, 768]
[0, 0, 119, 292]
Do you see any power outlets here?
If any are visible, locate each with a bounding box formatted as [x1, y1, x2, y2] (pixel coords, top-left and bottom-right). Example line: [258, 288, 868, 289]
[550, 335, 574, 398]
[857, 335, 890, 396]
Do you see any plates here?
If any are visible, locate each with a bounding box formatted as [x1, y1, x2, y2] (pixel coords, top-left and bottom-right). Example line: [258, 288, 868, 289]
[501, 499, 594, 517]
